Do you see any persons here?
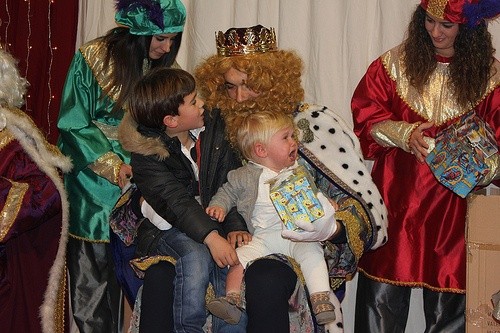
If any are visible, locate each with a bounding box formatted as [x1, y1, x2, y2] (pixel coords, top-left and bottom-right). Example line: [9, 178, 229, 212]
[0, 44, 74, 333]
[350, 0, 500, 333]
[54, 0, 186, 333]
[128, 25, 389, 333]
[119, 69, 251, 332]
[205, 112, 337, 326]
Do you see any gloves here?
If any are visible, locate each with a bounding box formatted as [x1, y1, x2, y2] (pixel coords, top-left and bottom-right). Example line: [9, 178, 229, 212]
[281, 192, 338, 242]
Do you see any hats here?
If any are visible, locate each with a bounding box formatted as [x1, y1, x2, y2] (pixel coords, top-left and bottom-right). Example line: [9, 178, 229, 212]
[419, 0, 499, 27]
[215, 25, 280, 57]
[114, 0, 186, 35]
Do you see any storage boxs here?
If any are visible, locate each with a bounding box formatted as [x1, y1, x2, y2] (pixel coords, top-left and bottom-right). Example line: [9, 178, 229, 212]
[264, 165, 326, 232]
[465, 183, 500, 333]
[425, 113, 499, 198]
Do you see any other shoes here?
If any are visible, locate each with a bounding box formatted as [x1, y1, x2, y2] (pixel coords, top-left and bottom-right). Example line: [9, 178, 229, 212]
[312, 301, 336, 324]
[208, 298, 244, 324]
